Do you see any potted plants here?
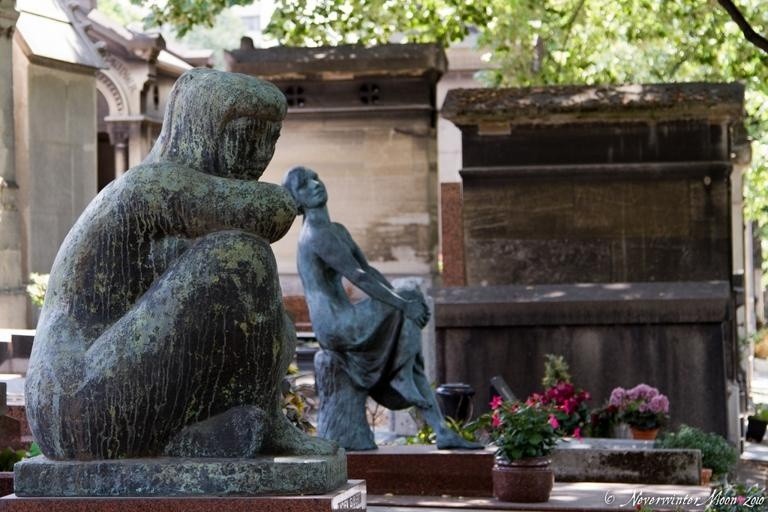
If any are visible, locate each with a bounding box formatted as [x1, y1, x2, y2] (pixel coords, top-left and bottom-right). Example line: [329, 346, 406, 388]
[745, 402, 767, 442]
[662, 427, 742, 485]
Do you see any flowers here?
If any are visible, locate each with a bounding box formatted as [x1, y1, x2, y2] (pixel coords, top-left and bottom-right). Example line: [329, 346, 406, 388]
[480, 352, 672, 459]
[24, 270, 51, 311]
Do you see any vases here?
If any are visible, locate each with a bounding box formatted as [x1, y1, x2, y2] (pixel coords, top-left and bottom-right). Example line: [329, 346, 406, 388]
[491, 457, 554, 503]
[434, 382, 476, 429]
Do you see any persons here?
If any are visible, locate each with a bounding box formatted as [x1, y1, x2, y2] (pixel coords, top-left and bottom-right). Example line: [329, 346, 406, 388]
[25, 68, 339, 460]
[284, 166, 485, 451]
